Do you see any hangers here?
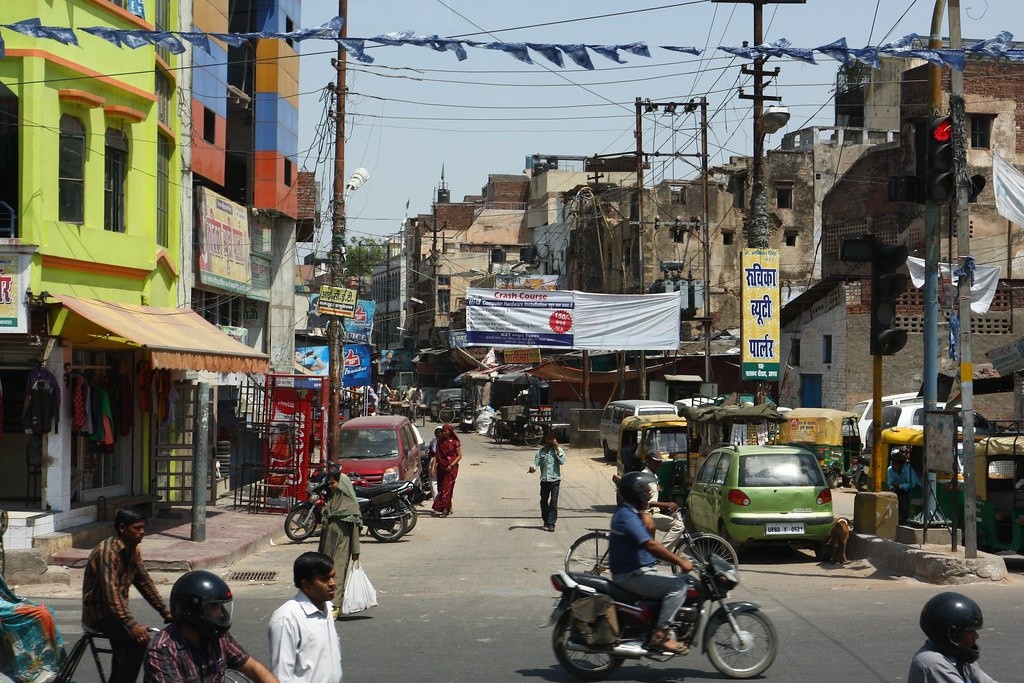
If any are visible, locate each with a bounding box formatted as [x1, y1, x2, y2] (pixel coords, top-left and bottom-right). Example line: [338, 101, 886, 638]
[31, 363, 171, 384]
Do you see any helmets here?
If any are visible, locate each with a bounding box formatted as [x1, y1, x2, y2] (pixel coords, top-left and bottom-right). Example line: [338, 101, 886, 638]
[169, 571, 234, 638]
[618, 472, 657, 509]
[920, 591, 983, 663]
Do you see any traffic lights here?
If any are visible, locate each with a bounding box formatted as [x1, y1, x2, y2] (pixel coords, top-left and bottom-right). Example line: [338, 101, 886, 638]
[968, 172, 987, 206]
[917, 110, 959, 206]
[839, 235, 876, 264]
[870, 243, 912, 358]
[888, 174, 920, 201]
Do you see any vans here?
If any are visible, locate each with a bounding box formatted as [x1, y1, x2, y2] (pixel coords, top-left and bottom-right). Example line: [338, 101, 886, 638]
[334, 415, 424, 503]
[601, 399, 679, 462]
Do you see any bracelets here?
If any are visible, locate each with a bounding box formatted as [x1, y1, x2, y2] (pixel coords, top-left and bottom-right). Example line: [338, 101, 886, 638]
[313, 355, 317, 360]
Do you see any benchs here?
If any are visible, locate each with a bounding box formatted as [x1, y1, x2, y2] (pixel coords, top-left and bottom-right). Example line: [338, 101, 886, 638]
[98, 495, 162, 521]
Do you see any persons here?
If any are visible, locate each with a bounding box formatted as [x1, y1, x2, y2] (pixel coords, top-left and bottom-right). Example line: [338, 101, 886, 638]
[381, 350, 405, 369]
[907, 591, 1001, 683]
[143, 569, 281, 683]
[639, 451, 685, 551]
[608, 472, 696, 655]
[408, 384, 423, 421]
[886, 451, 923, 491]
[0, 508, 71, 683]
[401, 383, 414, 400]
[79, 509, 172, 683]
[367, 382, 392, 414]
[308, 296, 332, 329]
[527, 431, 565, 531]
[294, 349, 325, 375]
[317, 471, 363, 622]
[427, 424, 463, 517]
[266, 551, 343, 683]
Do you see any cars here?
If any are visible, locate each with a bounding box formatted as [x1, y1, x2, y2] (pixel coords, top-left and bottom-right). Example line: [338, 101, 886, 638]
[844, 392, 970, 453]
[688, 441, 837, 560]
[431, 387, 466, 422]
[864, 403, 1008, 453]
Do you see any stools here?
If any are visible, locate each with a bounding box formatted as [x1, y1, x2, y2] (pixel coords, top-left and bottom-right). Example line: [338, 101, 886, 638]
[53, 624, 114, 683]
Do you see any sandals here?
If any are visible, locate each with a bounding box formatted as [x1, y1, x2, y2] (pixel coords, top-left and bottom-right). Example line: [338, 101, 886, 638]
[651, 634, 689, 655]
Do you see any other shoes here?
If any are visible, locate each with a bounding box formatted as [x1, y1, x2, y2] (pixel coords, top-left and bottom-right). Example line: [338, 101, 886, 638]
[333, 607, 340, 621]
[544, 521, 554, 532]
[440, 508, 450, 518]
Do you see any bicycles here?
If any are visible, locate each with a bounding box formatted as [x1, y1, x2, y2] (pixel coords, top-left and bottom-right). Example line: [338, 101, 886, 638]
[438, 397, 463, 424]
[495, 413, 546, 448]
[54, 623, 252, 682]
[565, 505, 740, 593]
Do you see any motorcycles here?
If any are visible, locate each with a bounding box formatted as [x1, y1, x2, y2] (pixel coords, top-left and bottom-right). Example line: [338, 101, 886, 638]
[282, 460, 425, 544]
[537, 557, 780, 680]
[611, 413, 694, 509]
[684, 393, 1024, 558]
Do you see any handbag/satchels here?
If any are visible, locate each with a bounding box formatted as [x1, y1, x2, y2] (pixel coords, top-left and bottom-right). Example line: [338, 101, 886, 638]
[342, 554, 379, 615]
[570, 594, 620, 646]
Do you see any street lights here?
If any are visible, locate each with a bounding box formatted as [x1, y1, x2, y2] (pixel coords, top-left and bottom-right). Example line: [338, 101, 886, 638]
[749, 103, 791, 416]
[322, 164, 371, 470]
[410, 297, 437, 331]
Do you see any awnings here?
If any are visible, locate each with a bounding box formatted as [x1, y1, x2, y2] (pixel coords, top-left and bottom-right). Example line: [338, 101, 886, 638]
[50, 291, 270, 374]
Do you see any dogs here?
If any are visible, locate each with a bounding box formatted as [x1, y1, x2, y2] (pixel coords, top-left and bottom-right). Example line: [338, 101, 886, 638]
[827, 517, 852, 567]
